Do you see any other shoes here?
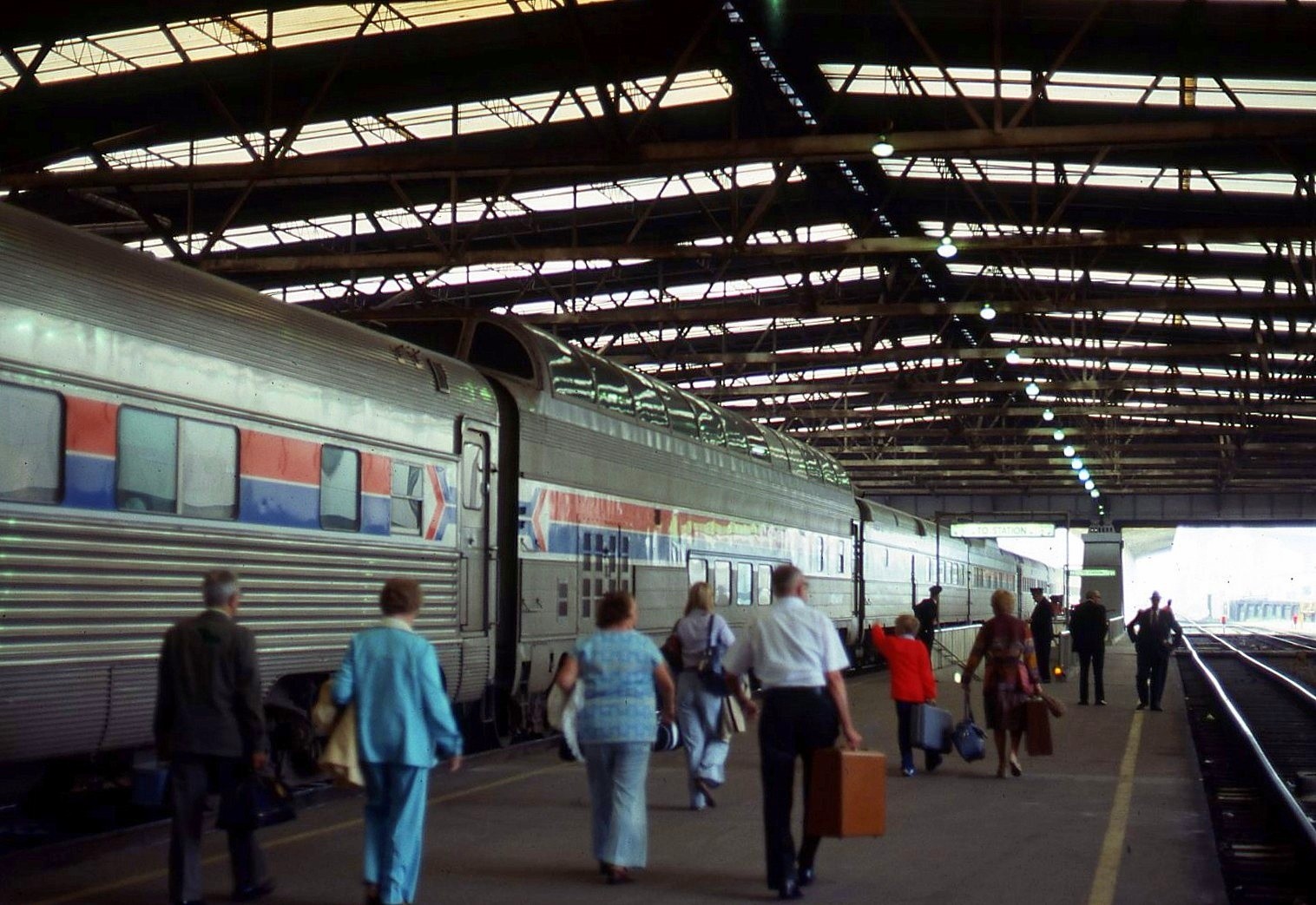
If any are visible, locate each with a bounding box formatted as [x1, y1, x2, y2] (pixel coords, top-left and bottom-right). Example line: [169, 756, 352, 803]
[1077, 700, 1088, 705]
[1150, 705, 1163, 712]
[904, 767, 917, 777]
[996, 765, 1009, 778]
[694, 777, 717, 807]
[799, 867, 816, 884]
[1136, 702, 1148, 710]
[1095, 699, 1106, 705]
[1009, 753, 1022, 777]
[232, 875, 276, 905]
[927, 755, 942, 772]
[778, 877, 804, 900]
[599, 862, 634, 886]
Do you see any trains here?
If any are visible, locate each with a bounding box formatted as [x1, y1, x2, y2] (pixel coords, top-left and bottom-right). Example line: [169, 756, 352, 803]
[0, 169, 1064, 807]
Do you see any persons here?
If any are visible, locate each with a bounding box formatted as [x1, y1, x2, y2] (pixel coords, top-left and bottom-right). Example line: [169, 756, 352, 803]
[557, 591, 676, 883]
[153, 568, 276, 905]
[871, 614, 943, 776]
[332, 578, 463, 905]
[721, 564, 863, 900]
[1070, 590, 1112, 704]
[914, 586, 942, 656]
[1127, 591, 1183, 713]
[962, 589, 1042, 780]
[666, 582, 737, 813]
[1027, 587, 1055, 684]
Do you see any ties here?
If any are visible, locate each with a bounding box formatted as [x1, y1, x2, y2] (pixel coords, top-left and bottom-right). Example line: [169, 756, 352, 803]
[1153, 611, 1158, 625]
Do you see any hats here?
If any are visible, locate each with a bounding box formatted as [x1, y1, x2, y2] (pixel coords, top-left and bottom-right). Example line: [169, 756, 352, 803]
[1150, 592, 1162, 602]
[1030, 587, 1044, 595]
[929, 585, 941, 594]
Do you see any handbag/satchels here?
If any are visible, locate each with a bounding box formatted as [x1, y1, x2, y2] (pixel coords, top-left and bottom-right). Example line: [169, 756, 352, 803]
[954, 689, 988, 763]
[1036, 688, 1067, 717]
[699, 615, 733, 696]
[238, 763, 298, 828]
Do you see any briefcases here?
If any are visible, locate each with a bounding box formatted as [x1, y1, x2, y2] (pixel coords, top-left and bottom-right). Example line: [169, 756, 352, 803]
[809, 743, 888, 839]
[910, 700, 953, 755]
[1024, 692, 1054, 757]
